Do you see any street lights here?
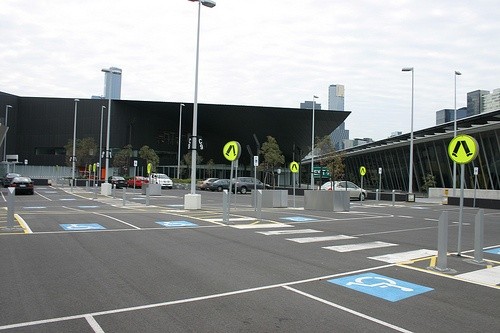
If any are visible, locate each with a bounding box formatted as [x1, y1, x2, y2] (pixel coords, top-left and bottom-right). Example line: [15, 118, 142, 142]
[310, 95, 319, 190]
[98, 106, 106, 180]
[176, 103, 186, 179]
[453, 70, 463, 197]
[3, 104, 13, 161]
[185, 0, 217, 194]
[71, 98, 79, 179]
[402, 66, 415, 193]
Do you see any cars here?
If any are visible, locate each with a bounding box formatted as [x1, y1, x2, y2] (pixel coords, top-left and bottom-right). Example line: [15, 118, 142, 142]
[8, 176, 35, 195]
[101, 175, 128, 189]
[126, 175, 149, 189]
[318, 180, 368, 201]
[0, 172, 23, 188]
[209, 179, 230, 192]
[197, 177, 220, 191]
[151, 173, 174, 190]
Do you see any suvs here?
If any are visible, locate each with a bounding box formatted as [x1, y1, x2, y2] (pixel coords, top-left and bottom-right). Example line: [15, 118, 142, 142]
[229, 176, 273, 194]
